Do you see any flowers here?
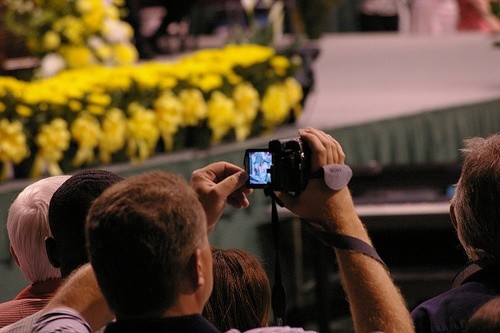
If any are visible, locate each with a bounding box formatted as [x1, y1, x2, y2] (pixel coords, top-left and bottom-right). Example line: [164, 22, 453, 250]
[0, 0, 306, 183]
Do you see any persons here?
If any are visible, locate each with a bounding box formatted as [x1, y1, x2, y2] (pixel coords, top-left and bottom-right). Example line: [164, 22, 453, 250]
[202, 247, 272, 332]
[410, 132, 500, 332]
[31, 126, 414, 333]
[7, 174, 72, 301]
[0, 168, 126, 333]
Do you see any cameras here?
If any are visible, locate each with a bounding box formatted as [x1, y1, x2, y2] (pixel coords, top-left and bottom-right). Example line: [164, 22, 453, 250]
[243, 137, 310, 193]
